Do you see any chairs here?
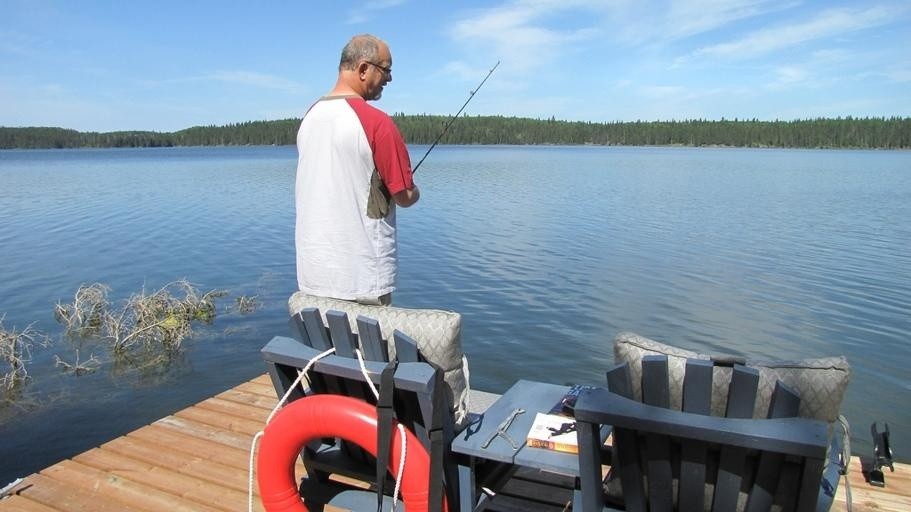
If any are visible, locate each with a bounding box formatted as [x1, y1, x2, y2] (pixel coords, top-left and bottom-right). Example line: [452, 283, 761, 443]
[260, 292, 518, 512]
[571, 333, 850, 512]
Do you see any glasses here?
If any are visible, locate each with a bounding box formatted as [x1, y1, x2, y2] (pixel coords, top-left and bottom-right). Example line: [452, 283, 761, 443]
[364, 60, 393, 76]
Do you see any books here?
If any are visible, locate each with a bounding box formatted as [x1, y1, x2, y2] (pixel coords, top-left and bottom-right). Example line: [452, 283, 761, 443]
[526, 412, 614, 455]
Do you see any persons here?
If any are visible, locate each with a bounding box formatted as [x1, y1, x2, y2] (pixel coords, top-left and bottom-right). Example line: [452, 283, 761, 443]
[294, 34, 420, 305]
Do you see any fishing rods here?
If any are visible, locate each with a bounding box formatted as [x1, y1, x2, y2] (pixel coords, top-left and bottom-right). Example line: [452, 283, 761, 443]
[409, 60, 502, 174]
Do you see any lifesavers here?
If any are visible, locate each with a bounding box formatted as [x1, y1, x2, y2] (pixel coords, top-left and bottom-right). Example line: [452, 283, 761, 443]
[257, 394, 447, 512]
